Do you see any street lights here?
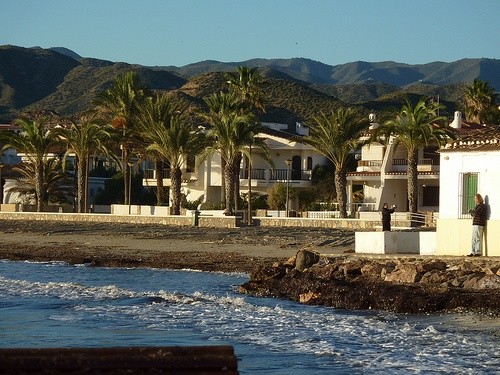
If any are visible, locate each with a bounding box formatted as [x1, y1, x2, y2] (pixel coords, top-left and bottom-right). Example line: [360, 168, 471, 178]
[285, 159, 294, 217]
[127, 161, 134, 214]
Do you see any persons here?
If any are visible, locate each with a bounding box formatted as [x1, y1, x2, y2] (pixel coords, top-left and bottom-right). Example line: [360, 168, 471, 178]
[382, 202, 398, 231]
[467, 193, 488, 258]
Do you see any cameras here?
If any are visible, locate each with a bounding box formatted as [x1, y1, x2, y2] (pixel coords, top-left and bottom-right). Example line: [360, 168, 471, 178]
[393, 204, 397, 207]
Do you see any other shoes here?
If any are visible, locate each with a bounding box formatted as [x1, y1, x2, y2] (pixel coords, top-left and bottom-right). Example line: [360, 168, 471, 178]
[467, 253, 473, 257]
[474, 254, 481, 257]
